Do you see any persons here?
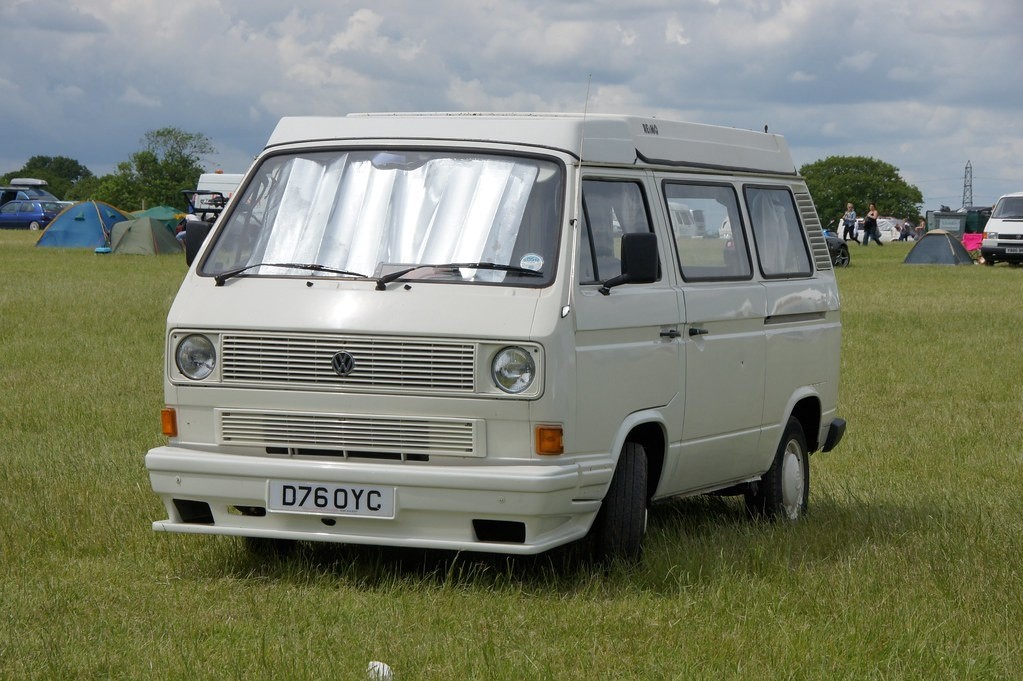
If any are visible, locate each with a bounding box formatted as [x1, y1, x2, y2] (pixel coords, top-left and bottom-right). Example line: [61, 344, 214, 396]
[863, 204, 884, 246]
[915, 217, 926, 240]
[891, 225, 902, 241]
[899, 219, 909, 241]
[840, 203, 861, 244]
[177, 214, 199, 247]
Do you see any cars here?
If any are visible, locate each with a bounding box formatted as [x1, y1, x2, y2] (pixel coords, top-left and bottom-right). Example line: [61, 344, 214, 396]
[718, 215, 740, 267]
[823, 230, 850, 270]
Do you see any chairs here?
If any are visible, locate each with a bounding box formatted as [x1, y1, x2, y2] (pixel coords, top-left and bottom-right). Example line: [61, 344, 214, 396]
[962, 233, 983, 264]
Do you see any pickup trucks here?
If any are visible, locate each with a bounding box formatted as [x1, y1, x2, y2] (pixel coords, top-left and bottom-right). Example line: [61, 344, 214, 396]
[0, 177, 81, 232]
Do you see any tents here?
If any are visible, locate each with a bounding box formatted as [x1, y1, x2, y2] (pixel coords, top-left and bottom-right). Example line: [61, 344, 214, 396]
[904, 229, 974, 264]
[837, 218, 913, 242]
[104, 217, 183, 255]
[132, 205, 188, 236]
[34, 200, 135, 248]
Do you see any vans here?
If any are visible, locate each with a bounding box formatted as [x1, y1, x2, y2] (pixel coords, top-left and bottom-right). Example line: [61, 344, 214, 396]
[668, 201, 699, 239]
[978, 191, 1023, 268]
[193, 169, 273, 226]
[835, 218, 908, 243]
[142, 112, 848, 563]
